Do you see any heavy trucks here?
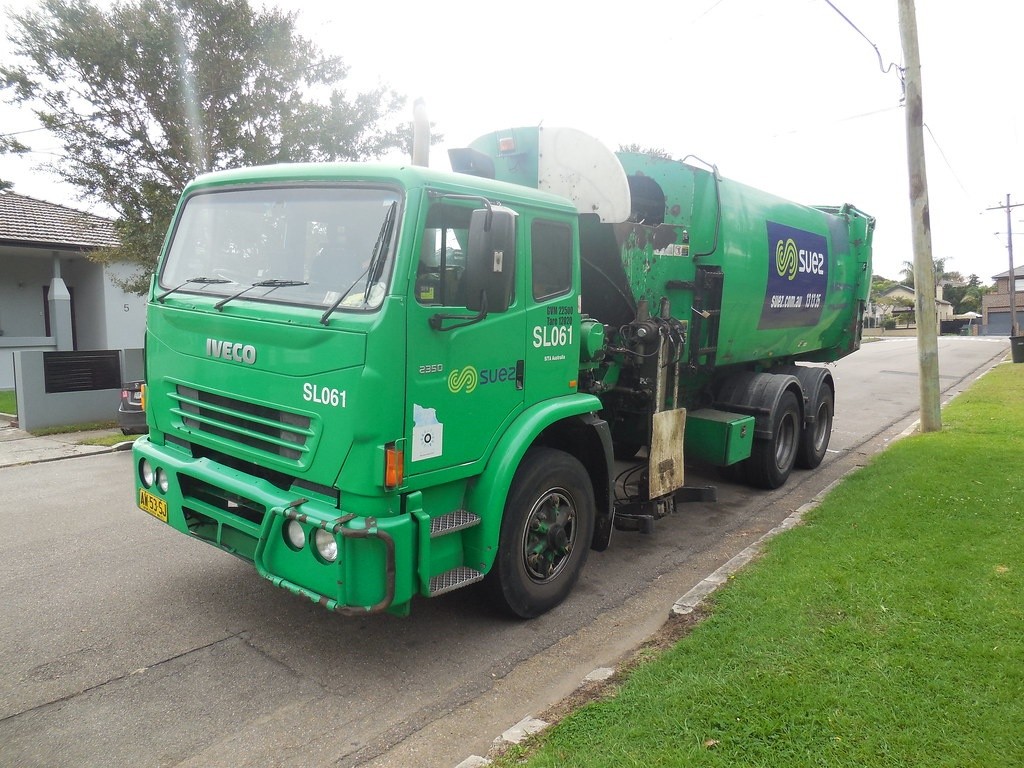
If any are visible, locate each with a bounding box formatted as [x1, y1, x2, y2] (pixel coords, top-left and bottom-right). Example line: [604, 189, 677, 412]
[129, 128, 879, 618]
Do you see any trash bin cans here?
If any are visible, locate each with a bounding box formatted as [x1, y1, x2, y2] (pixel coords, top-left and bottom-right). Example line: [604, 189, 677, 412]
[1008, 335, 1024, 363]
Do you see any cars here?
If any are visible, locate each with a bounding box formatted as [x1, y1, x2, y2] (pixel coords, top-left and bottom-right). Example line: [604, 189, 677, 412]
[120, 380, 148, 436]
